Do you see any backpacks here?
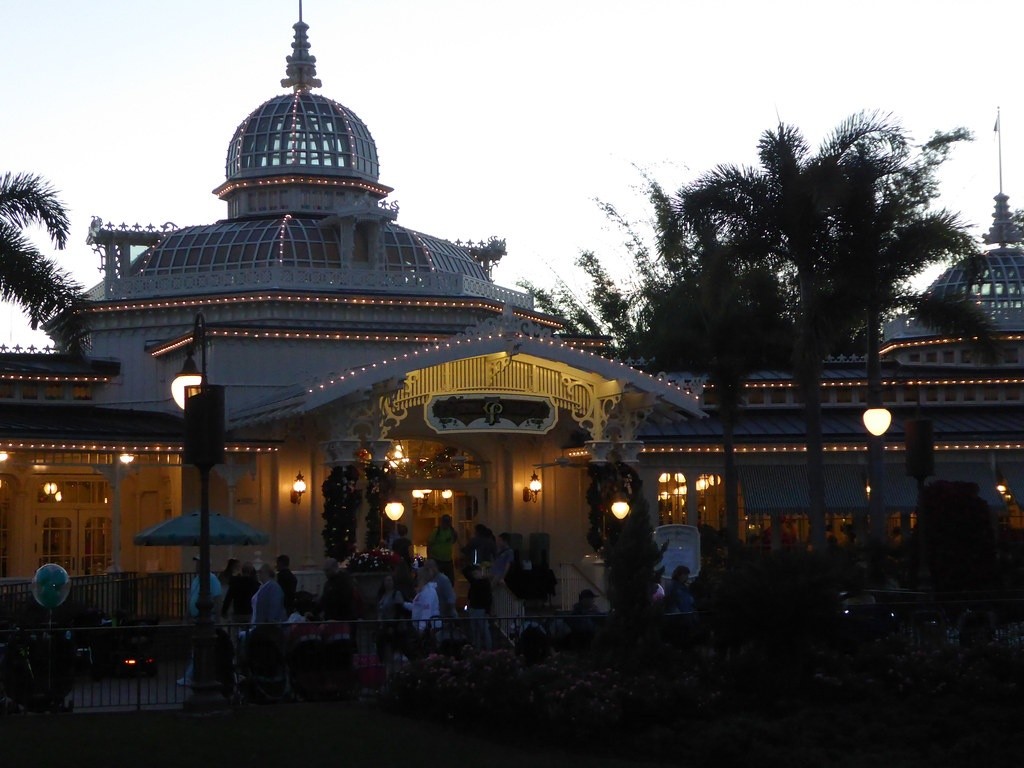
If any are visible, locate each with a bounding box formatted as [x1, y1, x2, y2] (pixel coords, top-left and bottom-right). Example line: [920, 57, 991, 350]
[520, 620, 550, 664]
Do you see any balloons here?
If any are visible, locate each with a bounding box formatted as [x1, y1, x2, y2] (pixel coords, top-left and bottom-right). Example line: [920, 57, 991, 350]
[31, 564, 71, 610]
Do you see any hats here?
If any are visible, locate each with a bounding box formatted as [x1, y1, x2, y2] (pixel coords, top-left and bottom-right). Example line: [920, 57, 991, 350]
[193, 555, 212, 564]
[443, 515, 450, 522]
[481, 561, 491, 568]
[579, 590, 599, 600]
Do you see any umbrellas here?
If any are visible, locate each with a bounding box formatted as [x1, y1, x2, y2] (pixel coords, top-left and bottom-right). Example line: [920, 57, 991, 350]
[133, 511, 269, 546]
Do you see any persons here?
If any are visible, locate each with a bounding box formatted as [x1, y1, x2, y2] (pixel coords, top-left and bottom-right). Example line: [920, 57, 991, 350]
[186, 514, 907, 666]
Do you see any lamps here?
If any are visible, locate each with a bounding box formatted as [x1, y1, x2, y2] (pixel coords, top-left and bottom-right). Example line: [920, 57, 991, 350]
[292, 469, 306, 504]
[523, 470, 542, 503]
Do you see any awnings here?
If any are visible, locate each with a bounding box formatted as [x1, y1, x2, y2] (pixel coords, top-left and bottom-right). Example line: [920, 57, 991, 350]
[740, 459, 1024, 516]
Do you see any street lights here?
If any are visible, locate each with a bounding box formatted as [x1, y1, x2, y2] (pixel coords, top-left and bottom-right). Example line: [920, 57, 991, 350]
[170, 313, 229, 714]
